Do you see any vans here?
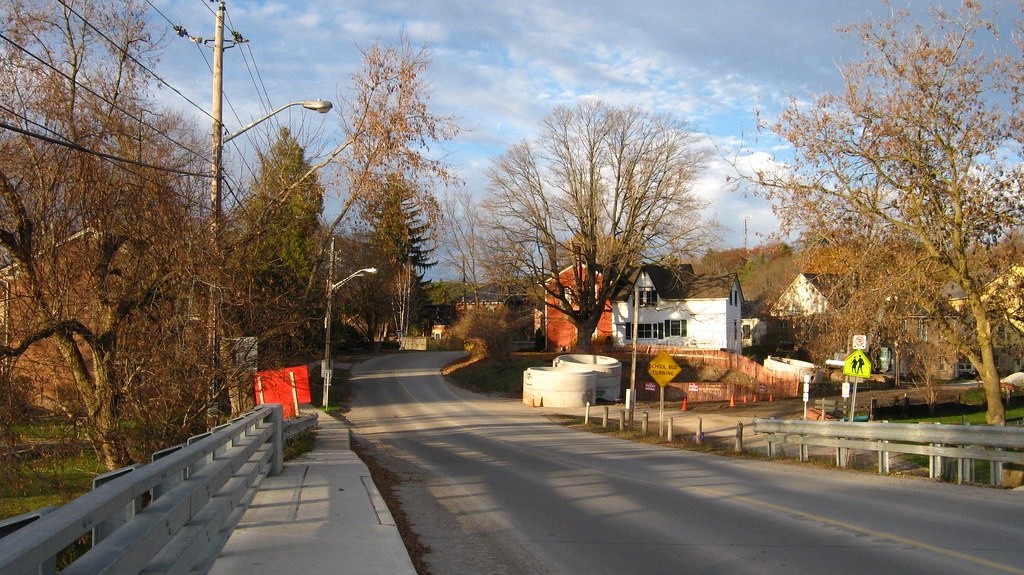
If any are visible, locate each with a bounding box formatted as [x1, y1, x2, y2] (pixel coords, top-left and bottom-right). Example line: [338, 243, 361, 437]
[959, 363, 976, 375]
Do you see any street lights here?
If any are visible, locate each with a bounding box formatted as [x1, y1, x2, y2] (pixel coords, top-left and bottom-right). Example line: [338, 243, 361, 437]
[744, 394, 748, 403]
[206, 98, 336, 433]
[322, 267, 378, 406]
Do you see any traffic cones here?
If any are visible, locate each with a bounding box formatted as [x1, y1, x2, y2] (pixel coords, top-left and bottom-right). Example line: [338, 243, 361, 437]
[530, 395, 536, 409]
[680, 397, 689, 412]
[752, 394, 758, 403]
[729, 394, 737, 408]
[768, 393, 774, 402]
